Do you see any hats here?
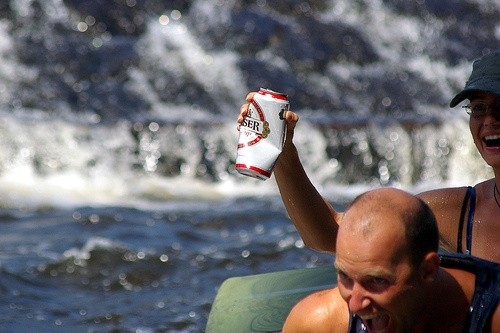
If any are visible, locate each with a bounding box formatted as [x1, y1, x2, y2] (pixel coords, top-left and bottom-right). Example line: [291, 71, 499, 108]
[449, 51, 500, 108]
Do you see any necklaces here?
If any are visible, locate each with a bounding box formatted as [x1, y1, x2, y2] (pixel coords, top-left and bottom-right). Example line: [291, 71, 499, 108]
[494, 183, 500, 208]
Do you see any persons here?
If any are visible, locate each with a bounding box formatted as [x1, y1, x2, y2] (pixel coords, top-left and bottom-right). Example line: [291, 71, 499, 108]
[281, 187, 470, 333]
[236, 51, 500, 333]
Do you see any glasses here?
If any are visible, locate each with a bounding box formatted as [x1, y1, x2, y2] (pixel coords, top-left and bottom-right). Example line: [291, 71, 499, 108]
[462, 103, 500, 116]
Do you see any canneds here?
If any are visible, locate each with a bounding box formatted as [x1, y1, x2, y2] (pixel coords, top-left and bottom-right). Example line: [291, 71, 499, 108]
[235, 88, 290, 180]
